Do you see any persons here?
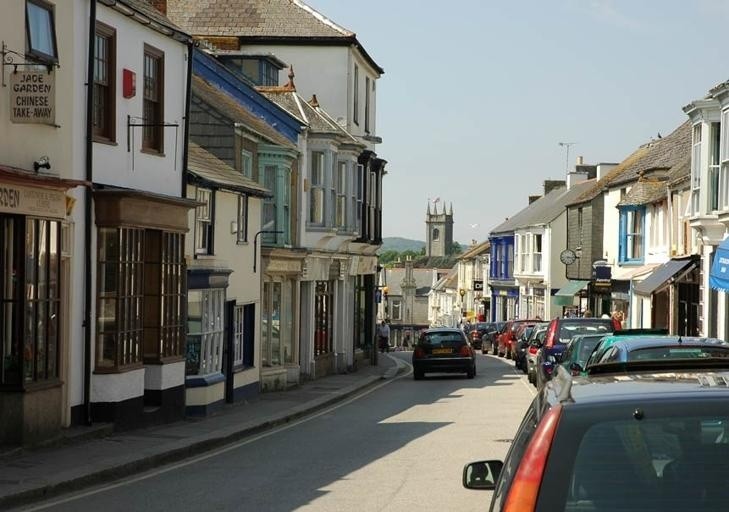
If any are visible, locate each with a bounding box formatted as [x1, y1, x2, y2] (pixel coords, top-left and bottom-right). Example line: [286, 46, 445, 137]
[583, 310, 593, 318]
[378, 320, 391, 354]
[611, 310, 623, 330]
[400, 334, 410, 351]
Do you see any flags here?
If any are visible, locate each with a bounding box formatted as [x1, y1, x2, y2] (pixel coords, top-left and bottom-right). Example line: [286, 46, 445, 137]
[594, 266, 612, 288]
[9, 70, 57, 124]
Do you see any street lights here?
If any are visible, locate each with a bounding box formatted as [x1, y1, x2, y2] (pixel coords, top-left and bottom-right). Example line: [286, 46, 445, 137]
[383, 287, 390, 325]
[459, 290, 465, 329]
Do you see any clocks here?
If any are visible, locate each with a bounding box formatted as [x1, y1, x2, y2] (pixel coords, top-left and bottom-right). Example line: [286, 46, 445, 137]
[560, 249, 577, 265]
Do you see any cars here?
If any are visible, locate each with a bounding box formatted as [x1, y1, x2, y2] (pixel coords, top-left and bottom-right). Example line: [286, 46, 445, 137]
[411, 328, 476, 381]
[523, 324, 551, 386]
[546, 331, 612, 382]
[569, 333, 728, 377]
[463, 356, 727, 512]
[463, 317, 542, 368]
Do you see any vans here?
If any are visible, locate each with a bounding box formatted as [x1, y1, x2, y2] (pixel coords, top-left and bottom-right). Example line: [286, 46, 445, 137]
[536, 317, 621, 392]
